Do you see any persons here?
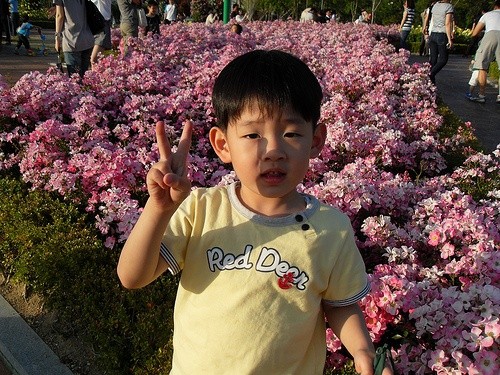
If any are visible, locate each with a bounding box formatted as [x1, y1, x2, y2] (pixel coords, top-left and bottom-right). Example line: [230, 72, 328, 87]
[300, 8, 340, 24]
[140, 0, 161, 39]
[53, 0, 94, 80]
[0, 0, 20, 45]
[231, 24, 242, 35]
[205, 4, 249, 25]
[419, 0, 455, 56]
[396, 0, 415, 54]
[91, 0, 112, 72]
[464, 0, 500, 102]
[428, 0, 454, 92]
[355, 7, 371, 25]
[164, 0, 178, 25]
[117, 0, 143, 57]
[117, 50, 392, 375]
[15, 14, 39, 55]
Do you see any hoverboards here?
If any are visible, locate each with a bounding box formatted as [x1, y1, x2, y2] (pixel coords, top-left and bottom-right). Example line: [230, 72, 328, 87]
[24, 27, 48, 57]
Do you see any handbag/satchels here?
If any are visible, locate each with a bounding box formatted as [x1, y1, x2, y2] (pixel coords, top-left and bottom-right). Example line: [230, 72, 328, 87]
[138, 9, 147, 26]
[86, 1, 105, 35]
[49, 53, 70, 78]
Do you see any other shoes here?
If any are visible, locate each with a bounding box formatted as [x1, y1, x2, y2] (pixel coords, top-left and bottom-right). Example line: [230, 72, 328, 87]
[470, 95, 487, 102]
[464, 92, 477, 99]
